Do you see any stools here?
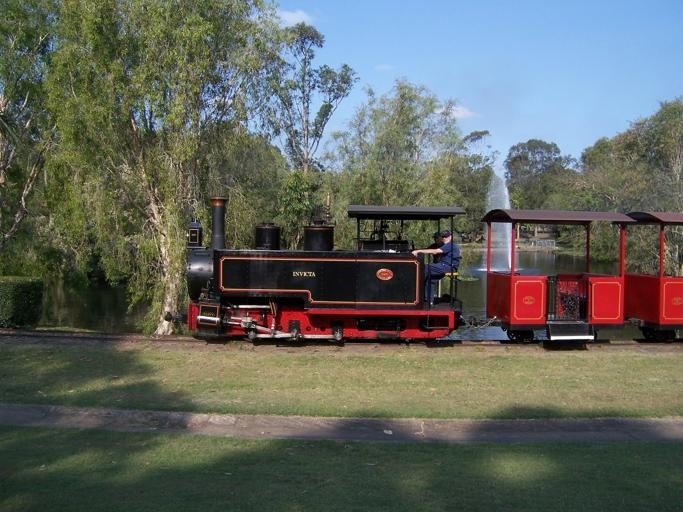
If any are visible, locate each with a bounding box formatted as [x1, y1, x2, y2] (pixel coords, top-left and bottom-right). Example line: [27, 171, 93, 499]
[444, 272, 458, 304]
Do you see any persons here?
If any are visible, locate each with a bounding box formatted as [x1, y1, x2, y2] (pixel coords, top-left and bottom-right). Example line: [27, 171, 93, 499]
[412, 230, 460, 305]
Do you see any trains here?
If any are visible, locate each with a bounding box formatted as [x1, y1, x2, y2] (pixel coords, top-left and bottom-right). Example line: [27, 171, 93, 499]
[163, 196, 683, 343]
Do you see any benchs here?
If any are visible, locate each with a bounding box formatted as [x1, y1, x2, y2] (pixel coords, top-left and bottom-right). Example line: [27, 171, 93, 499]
[557, 287, 585, 317]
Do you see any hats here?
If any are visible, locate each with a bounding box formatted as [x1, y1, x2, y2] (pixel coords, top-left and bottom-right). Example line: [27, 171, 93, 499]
[432, 232, 441, 238]
[441, 230, 451, 236]
